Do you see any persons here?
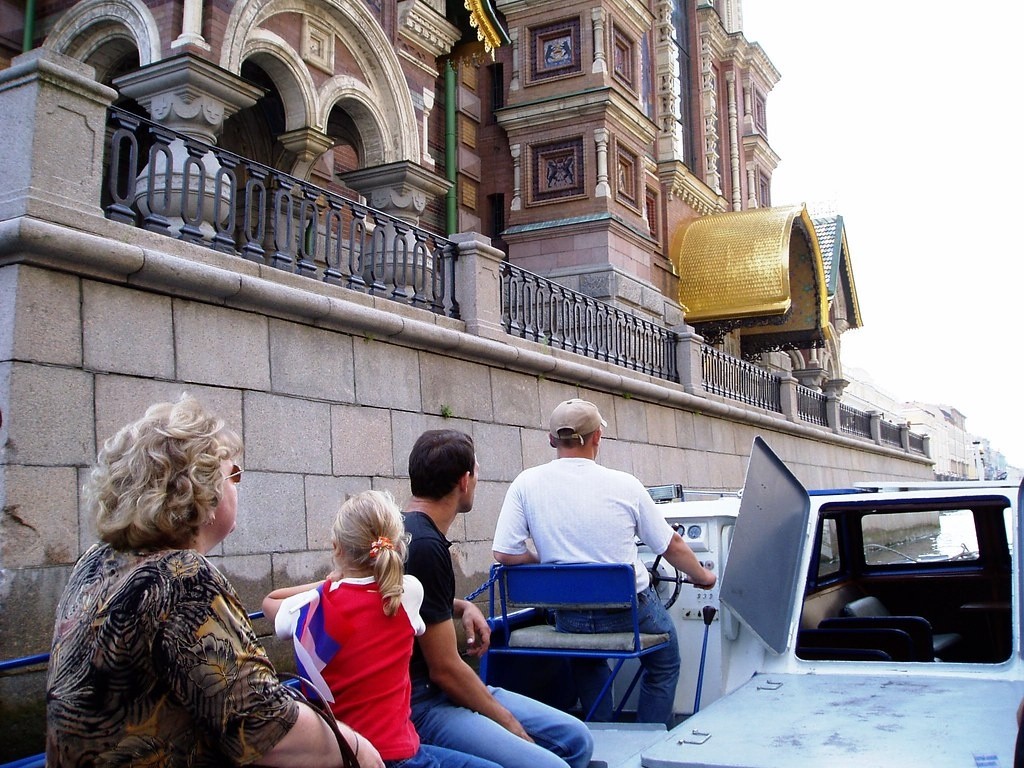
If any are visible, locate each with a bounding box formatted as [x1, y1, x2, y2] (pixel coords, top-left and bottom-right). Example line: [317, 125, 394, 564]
[399, 429, 594, 768]
[264, 492, 503, 768]
[492, 398, 716, 724]
[46, 398, 385, 768]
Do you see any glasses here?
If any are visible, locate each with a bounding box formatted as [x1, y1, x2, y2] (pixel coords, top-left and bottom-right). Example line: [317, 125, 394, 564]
[223, 464, 245, 483]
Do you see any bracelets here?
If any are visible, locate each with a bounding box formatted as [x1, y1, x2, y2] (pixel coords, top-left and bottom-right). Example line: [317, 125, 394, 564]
[353, 731, 359, 757]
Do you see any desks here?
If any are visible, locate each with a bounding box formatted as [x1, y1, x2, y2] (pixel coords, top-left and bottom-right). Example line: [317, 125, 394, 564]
[960, 600, 1012, 659]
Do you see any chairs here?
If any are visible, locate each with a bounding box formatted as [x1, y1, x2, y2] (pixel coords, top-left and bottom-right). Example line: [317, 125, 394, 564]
[479, 562, 672, 723]
[841, 597, 961, 663]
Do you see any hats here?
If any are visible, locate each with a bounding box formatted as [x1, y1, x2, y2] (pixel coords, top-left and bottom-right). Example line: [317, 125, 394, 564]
[550, 398, 608, 444]
